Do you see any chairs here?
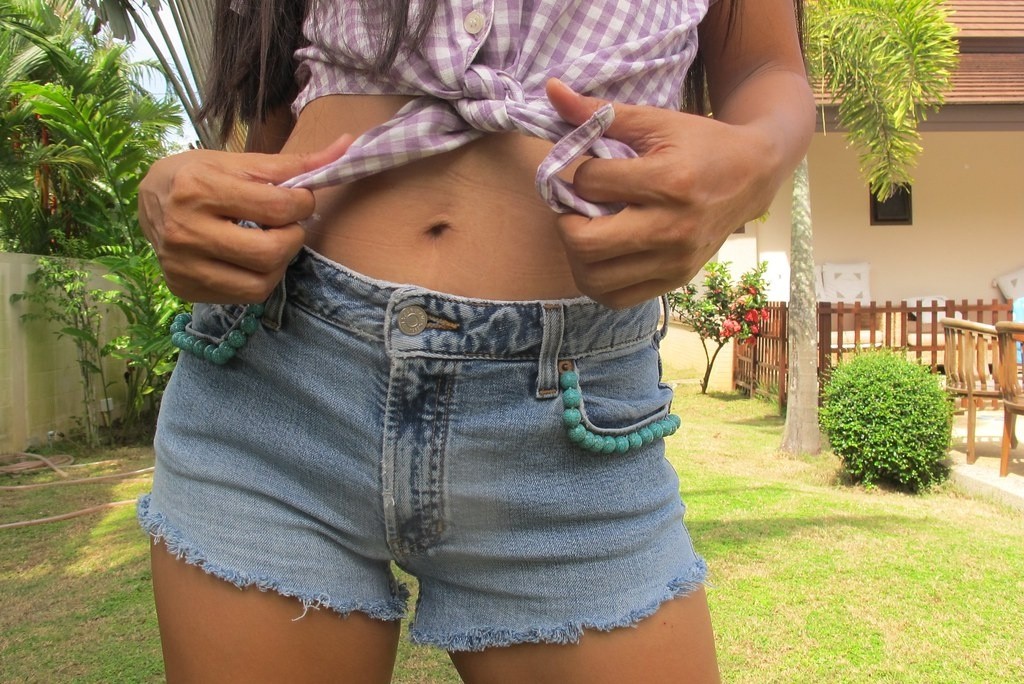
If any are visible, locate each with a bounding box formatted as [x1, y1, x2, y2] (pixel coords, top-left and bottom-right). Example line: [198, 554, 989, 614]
[935, 315, 1024, 478]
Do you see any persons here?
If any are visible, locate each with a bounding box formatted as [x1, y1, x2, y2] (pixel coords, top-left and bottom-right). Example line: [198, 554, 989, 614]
[136, 0, 818, 684]
